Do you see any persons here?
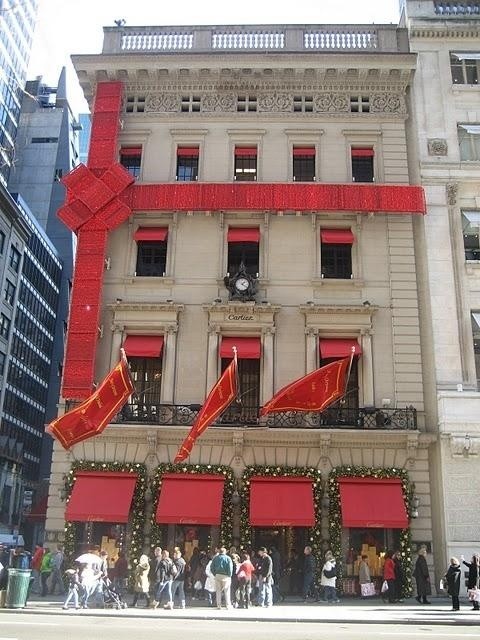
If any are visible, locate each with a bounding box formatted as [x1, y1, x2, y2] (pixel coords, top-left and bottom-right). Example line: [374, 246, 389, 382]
[319, 549, 339, 603]
[444, 557, 461, 610]
[394, 552, 404, 602]
[0, 543, 282, 609]
[414, 548, 430, 604]
[462, 556, 480, 610]
[285, 548, 304, 596]
[303, 546, 320, 602]
[358, 555, 372, 598]
[381, 551, 398, 602]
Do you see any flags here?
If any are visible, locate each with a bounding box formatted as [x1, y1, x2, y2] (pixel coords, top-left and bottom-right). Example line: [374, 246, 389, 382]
[175, 357, 238, 464]
[46, 355, 133, 449]
[260, 353, 351, 416]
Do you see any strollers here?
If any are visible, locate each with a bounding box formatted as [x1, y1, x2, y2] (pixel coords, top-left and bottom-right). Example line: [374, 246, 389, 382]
[97, 572, 127, 610]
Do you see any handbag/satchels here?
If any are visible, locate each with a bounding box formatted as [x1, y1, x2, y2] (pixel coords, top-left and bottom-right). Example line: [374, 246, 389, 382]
[359, 583, 376, 596]
[380, 579, 389, 592]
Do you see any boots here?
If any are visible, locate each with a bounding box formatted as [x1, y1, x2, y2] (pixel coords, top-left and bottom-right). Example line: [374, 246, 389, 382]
[164, 601, 174, 610]
[149, 599, 160, 608]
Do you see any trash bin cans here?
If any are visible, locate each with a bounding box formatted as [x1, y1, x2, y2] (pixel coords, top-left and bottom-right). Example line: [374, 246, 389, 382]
[4, 568, 35, 609]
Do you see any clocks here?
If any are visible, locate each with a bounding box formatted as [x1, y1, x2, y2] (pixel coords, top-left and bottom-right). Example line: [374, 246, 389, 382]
[235, 278, 249, 291]
